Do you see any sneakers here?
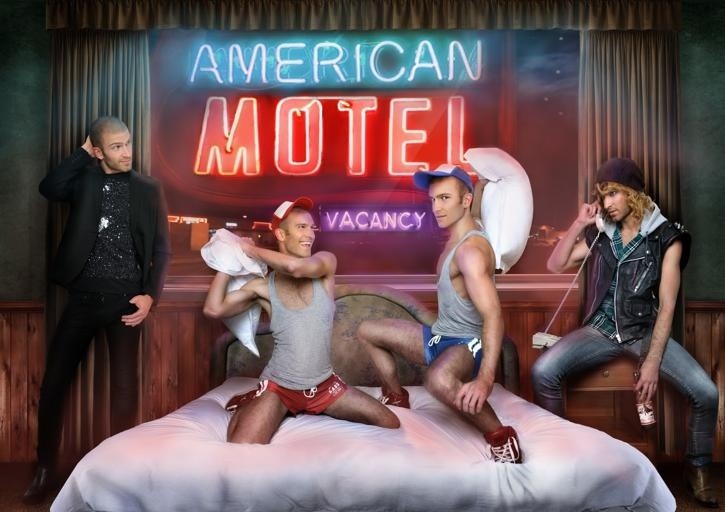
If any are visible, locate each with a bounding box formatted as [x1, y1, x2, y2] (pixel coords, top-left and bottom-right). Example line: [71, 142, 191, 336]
[482, 426, 522, 463]
[225, 387, 259, 411]
[378, 384, 411, 407]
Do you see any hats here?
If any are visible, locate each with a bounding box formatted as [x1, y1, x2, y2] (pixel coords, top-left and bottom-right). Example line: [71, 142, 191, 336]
[413, 164, 474, 193]
[595, 158, 644, 191]
[272, 196, 314, 228]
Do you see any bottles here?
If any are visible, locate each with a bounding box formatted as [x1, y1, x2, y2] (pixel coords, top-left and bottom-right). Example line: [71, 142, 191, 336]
[633, 372, 657, 430]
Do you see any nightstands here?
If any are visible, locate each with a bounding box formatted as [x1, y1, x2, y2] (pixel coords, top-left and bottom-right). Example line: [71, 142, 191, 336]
[563, 357, 674, 464]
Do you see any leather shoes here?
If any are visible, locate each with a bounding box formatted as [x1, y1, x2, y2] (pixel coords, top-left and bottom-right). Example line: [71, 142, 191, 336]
[684, 462, 718, 508]
[22, 465, 52, 505]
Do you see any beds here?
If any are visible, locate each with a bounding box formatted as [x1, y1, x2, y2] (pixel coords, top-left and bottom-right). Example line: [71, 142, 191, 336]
[50, 284, 676, 512]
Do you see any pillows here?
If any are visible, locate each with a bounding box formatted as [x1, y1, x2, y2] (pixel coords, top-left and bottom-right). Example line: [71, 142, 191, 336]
[464, 148, 534, 274]
[201, 228, 268, 358]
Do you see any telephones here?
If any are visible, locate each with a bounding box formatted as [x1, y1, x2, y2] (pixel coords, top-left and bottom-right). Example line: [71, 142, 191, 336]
[596, 212, 606, 232]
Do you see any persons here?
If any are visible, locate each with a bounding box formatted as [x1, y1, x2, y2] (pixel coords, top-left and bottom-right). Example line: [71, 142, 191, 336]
[530, 161, 720, 508]
[202, 196, 400, 445]
[23, 115, 173, 504]
[354, 166, 524, 465]
